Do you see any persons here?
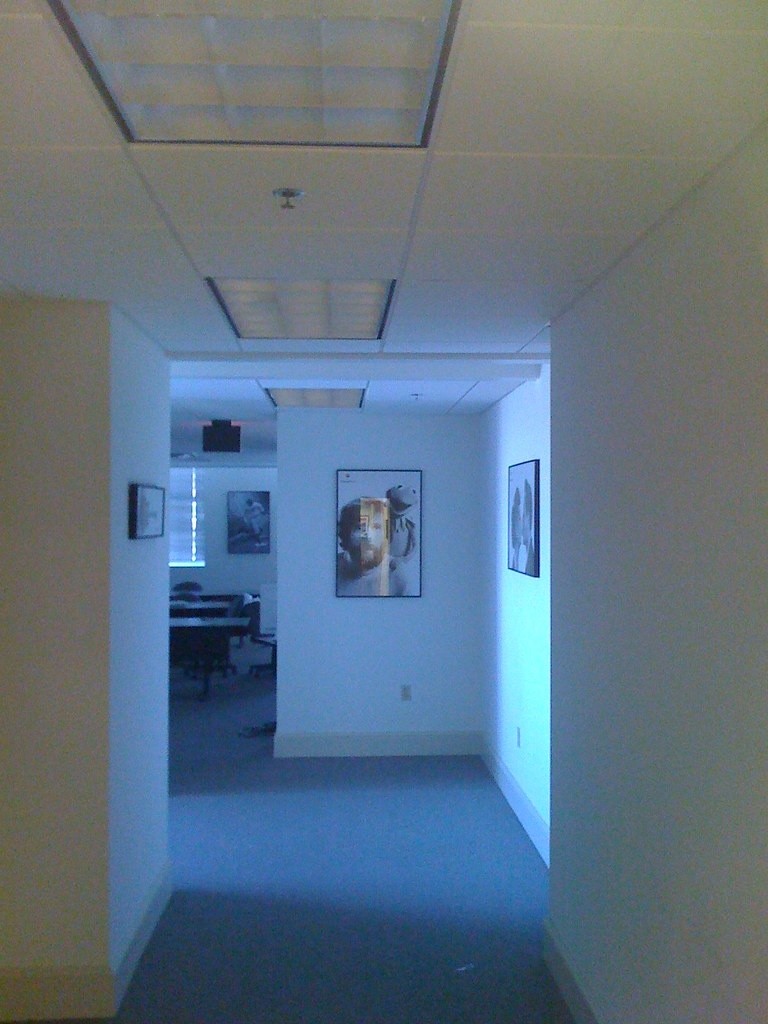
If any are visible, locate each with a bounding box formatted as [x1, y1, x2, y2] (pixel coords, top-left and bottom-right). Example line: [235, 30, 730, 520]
[242, 498, 267, 538]
[508, 479, 535, 575]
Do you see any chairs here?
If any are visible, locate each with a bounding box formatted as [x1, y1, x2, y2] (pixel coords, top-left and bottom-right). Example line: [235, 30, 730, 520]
[252, 599, 277, 678]
[216, 593, 249, 677]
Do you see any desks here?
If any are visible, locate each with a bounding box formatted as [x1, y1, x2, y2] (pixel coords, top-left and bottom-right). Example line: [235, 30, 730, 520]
[171, 601, 232, 614]
[168, 617, 251, 702]
[169, 589, 261, 600]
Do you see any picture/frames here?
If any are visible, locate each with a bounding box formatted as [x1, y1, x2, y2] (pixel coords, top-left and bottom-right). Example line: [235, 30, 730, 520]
[336, 469, 423, 598]
[507, 458, 541, 578]
[128, 484, 165, 540]
[226, 489, 274, 555]
